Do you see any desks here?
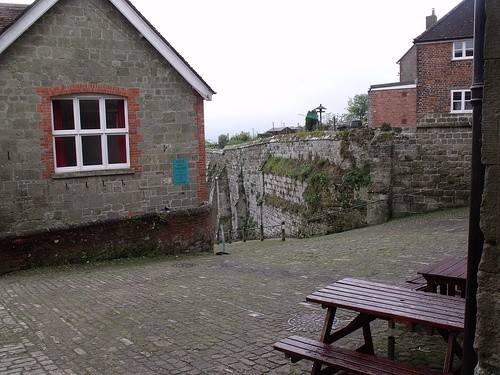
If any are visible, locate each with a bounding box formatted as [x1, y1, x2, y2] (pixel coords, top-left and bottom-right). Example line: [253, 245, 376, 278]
[416, 251, 469, 300]
[304, 277, 467, 375]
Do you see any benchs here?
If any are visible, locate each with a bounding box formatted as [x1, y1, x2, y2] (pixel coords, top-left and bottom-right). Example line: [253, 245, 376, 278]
[272, 334, 455, 375]
[394, 271, 429, 291]
